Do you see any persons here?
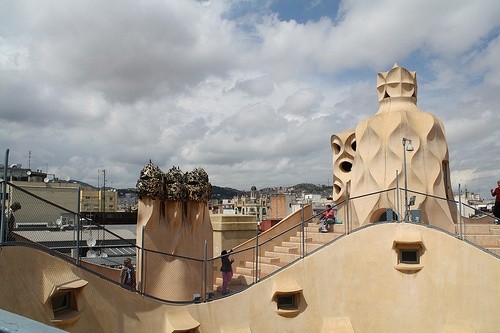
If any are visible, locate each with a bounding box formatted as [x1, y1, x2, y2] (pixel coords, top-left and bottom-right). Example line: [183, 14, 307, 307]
[316, 204, 335, 232]
[220, 249, 235, 295]
[119, 258, 136, 290]
[5, 202, 21, 246]
[490, 180, 500, 225]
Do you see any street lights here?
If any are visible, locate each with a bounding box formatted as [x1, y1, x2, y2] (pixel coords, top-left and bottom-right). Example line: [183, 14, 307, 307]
[402, 138, 417, 222]
